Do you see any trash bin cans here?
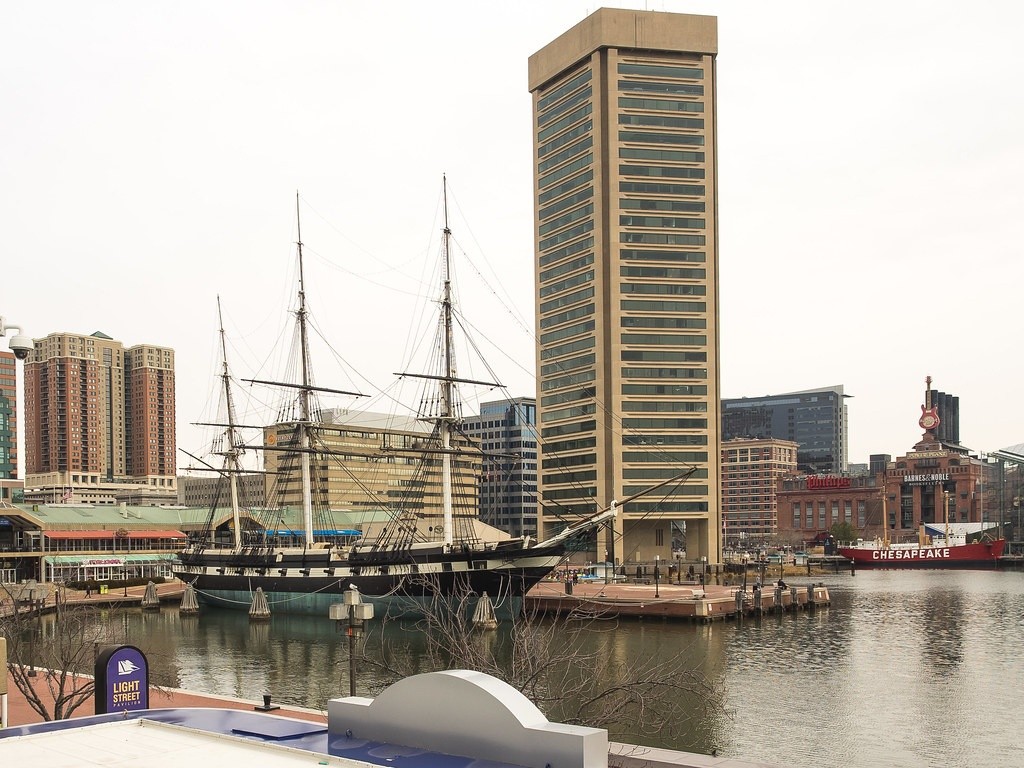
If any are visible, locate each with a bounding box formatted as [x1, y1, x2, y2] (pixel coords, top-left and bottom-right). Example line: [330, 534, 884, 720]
[98, 585, 108, 595]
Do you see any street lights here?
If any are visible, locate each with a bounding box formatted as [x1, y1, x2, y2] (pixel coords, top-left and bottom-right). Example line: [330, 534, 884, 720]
[701, 556, 707, 598]
[743, 550, 751, 593]
[122, 557, 130, 597]
[677, 551, 683, 584]
[604, 549, 609, 584]
[564, 557, 570, 594]
[653, 553, 660, 598]
[760, 549, 766, 588]
[778, 550, 784, 580]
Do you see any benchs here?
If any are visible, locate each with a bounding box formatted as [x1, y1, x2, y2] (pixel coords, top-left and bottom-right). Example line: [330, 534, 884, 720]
[730, 586, 749, 597]
[773, 583, 789, 592]
[691, 589, 710, 600]
[632, 578, 651, 585]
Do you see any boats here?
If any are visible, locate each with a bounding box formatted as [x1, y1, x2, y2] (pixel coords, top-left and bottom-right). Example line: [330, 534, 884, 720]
[835, 485, 1006, 570]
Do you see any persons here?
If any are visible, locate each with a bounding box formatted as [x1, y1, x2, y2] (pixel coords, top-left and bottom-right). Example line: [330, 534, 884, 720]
[84, 585, 91, 599]
[756, 574, 761, 590]
[699, 573, 703, 587]
[685, 572, 691, 581]
[778, 579, 787, 591]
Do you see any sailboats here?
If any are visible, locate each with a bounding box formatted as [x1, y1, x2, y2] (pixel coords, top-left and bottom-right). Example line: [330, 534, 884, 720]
[172, 170, 700, 629]
